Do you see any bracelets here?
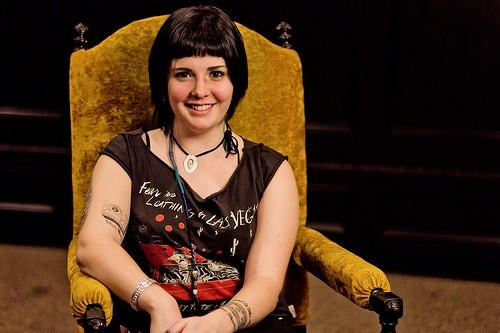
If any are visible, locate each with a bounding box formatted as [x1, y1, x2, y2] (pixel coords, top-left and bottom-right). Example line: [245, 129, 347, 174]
[130, 279, 160, 312]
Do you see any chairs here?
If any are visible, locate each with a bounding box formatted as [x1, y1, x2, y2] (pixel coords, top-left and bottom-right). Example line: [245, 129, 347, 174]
[69, 14, 404, 333]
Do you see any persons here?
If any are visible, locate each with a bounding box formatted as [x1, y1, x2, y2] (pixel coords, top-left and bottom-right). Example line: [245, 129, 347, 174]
[75, 5, 299, 333]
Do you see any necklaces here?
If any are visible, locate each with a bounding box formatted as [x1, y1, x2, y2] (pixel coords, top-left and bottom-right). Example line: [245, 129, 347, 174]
[170, 121, 239, 174]
[169, 136, 201, 317]
